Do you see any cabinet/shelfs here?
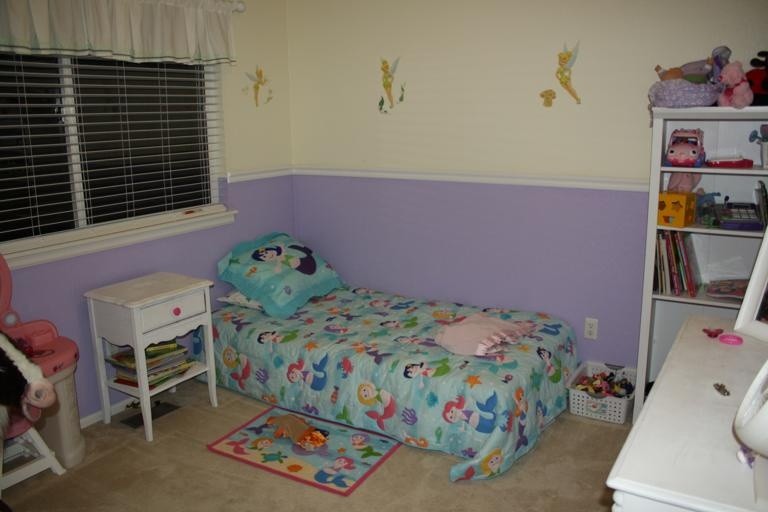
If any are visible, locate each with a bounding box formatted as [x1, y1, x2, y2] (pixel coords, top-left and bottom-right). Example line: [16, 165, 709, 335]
[632, 103, 768, 432]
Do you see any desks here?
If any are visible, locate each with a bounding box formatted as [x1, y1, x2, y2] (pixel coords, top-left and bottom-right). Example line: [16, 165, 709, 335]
[602, 312, 767, 512]
[83, 268, 217, 443]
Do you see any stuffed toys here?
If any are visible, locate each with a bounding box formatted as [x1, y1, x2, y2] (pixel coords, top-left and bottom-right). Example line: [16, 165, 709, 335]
[577, 370, 635, 398]
[0, 330, 56, 422]
[648, 46, 768, 128]
[267, 414, 329, 449]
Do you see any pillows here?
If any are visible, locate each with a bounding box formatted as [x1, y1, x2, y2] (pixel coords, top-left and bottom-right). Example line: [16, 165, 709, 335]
[214, 228, 346, 321]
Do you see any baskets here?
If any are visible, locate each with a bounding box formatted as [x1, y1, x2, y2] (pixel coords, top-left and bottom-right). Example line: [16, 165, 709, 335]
[564, 360, 636, 424]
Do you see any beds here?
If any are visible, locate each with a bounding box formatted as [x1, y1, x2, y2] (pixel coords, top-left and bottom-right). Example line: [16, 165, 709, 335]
[195, 284, 572, 470]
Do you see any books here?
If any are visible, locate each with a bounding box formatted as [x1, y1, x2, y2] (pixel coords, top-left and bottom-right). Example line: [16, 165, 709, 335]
[706, 279, 748, 299]
[653, 231, 697, 301]
[106, 341, 196, 393]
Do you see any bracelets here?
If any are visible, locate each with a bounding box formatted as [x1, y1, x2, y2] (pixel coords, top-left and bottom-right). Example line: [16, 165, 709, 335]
[719, 334, 743, 345]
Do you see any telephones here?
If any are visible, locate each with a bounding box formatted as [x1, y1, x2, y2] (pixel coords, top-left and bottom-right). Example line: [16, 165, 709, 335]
[717, 202, 763, 230]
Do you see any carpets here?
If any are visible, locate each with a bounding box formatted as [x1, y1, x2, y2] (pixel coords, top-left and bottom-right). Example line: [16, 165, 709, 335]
[205, 405, 402, 496]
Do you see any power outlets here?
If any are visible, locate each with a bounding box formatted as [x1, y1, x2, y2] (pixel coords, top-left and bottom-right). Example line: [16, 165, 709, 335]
[583, 317, 597, 340]
